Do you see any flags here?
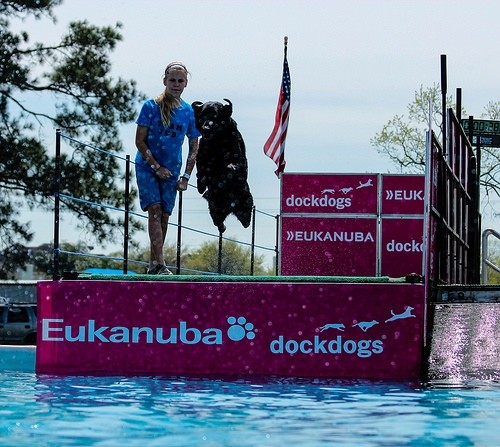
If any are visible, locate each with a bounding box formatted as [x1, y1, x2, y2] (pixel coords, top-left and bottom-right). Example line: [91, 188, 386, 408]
[264, 46, 291, 180]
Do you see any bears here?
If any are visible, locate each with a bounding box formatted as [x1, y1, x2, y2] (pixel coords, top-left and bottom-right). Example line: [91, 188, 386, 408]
[191, 94, 255, 232]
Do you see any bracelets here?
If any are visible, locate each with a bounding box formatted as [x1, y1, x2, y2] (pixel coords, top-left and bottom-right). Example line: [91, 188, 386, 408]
[155, 164, 160, 170]
[180, 172, 190, 180]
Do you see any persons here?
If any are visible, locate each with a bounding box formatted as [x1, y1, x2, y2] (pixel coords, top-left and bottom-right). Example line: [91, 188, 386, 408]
[135, 62, 200, 276]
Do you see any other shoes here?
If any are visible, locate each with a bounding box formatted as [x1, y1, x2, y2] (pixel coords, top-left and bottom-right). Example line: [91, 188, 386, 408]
[156, 266, 172, 275]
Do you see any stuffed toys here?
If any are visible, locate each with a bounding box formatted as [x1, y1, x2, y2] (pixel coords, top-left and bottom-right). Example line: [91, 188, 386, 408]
[191, 98, 253, 234]
